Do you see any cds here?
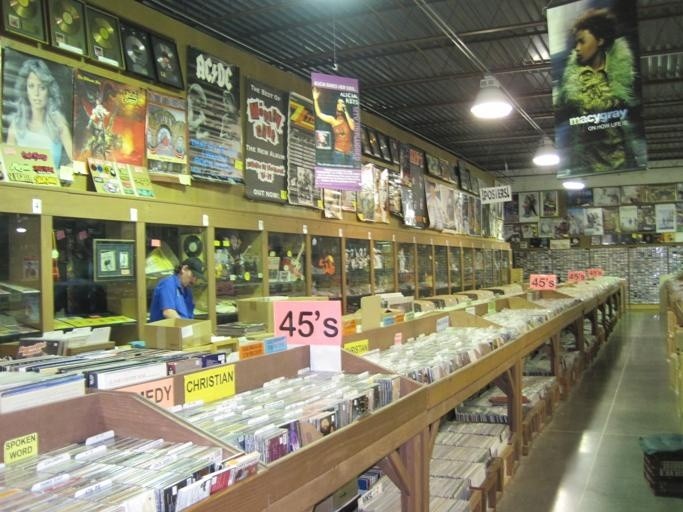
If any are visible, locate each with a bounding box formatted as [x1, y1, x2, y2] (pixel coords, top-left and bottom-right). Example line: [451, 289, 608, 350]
[10, 0, 176, 72]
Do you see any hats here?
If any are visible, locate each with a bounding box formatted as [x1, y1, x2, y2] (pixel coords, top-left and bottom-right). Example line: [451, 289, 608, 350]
[184, 257, 209, 283]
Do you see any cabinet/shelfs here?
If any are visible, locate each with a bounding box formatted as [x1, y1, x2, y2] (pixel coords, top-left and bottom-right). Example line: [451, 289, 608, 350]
[0, 182, 628, 512]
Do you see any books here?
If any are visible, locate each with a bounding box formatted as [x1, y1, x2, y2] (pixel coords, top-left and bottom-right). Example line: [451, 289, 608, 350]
[455, 340, 555, 425]
[2, 326, 288, 413]
[338, 421, 512, 508]
[2, 436, 262, 509]
[173, 370, 401, 464]
[366, 326, 539, 384]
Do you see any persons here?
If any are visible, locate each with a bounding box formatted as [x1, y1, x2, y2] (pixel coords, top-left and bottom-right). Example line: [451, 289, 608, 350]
[555, 9, 646, 176]
[311, 87, 356, 165]
[147, 256, 208, 321]
[425, 181, 445, 230]
[7, 58, 71, 170]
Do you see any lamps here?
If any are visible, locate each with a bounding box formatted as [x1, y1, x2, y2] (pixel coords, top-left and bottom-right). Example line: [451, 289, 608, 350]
[532, 133, 559, 167]
[562, 178, 584, 189]
[469, 74, 513, 119]
